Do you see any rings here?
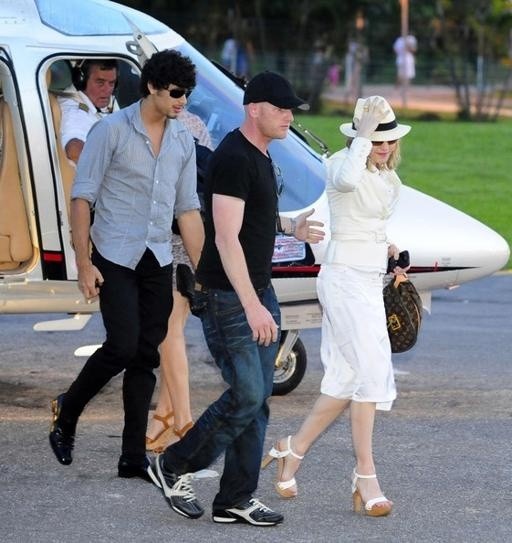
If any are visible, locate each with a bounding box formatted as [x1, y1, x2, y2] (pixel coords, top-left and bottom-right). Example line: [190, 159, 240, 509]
[363, 106, 368, 112]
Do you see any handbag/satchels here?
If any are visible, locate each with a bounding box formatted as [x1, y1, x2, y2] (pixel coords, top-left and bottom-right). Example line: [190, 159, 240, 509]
[382, 274, 422, 353]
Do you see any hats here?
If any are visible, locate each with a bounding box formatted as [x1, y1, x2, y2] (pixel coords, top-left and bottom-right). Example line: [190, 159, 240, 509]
[340, 95, 412, 141]
[244, 72, 310, 110]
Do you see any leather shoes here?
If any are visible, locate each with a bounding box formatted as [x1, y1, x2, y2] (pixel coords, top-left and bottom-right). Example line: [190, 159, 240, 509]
[50, 393, 75, 464]
[118, 456, 154, 484]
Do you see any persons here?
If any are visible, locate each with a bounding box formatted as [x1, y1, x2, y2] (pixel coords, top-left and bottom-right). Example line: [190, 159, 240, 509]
[328, 63, 344, 87]
[146, 108, 214, 454]
[53, 58, 120, 165]
[260, 95, 413, 519]
[341, 11, 369, 107]
[393, 33, 418, 101]
[221, 33, 247, 80]
[145, 70, 326, 528]
[48, 48, 207, 483]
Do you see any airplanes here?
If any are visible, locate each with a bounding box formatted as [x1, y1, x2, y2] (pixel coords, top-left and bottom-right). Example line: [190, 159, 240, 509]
[0, 0, 511, 400]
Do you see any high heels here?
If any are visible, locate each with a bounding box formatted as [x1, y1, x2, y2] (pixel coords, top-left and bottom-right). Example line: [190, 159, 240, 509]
[262, 435, 303, 497]
[351, 468, 391, 516]
[146, 411, 174, 451]
[154, 422, 194, 454]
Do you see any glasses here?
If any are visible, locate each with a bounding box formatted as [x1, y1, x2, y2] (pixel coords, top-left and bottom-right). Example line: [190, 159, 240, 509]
[164, 87, 192, 98]
[272, 161, 285, 197]
[371, 141, 397, 146]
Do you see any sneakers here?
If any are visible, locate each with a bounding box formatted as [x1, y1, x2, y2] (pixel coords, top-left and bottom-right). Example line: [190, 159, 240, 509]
[212, 499, 283, 526]
[146, 453, 204, 519]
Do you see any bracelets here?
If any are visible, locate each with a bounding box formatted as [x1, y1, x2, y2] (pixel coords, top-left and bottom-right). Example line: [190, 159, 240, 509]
[287, 217, 296, 237]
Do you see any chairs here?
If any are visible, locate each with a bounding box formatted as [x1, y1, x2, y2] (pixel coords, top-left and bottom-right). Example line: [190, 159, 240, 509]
[0, 96, 33, 272]
[46, 68, 94, 259]
